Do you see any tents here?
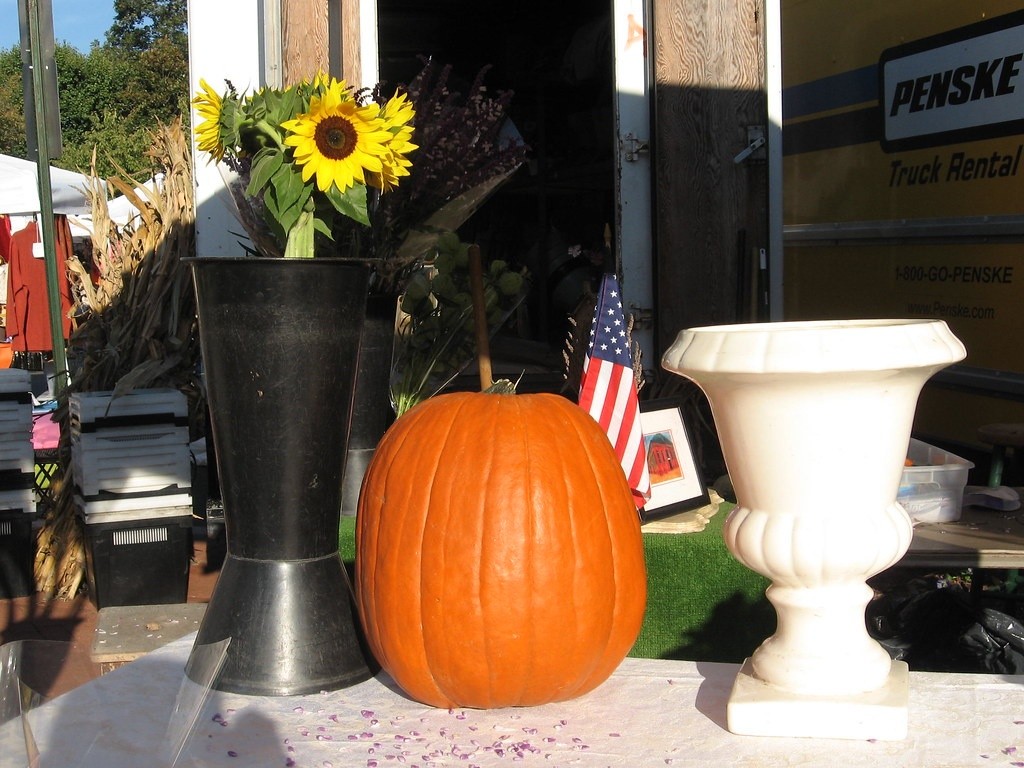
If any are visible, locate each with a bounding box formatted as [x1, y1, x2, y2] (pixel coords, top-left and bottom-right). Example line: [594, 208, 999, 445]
[66, 172, 165, 236]
[0, 153, 107, 237]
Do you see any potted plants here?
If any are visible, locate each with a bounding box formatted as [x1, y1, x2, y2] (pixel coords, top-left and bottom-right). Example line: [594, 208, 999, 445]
[182, 255, 380, 698]
[216, 54, 531, 516]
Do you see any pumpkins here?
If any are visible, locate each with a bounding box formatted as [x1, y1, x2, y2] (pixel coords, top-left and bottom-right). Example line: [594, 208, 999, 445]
[354, 378, 646, 711]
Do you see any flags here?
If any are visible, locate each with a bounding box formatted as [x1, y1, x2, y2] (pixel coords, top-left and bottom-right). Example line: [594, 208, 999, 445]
[577, 248, 651, 511]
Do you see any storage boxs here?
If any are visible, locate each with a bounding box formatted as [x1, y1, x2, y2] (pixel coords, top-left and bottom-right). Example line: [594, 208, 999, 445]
[896, 438, 975, 524]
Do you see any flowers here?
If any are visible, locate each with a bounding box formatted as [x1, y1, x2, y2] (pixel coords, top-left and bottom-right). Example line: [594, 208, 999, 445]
[192, 65, 420, 260]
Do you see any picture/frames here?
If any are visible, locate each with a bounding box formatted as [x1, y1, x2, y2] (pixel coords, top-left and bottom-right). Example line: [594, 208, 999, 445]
[637, 395, 711, 525]
